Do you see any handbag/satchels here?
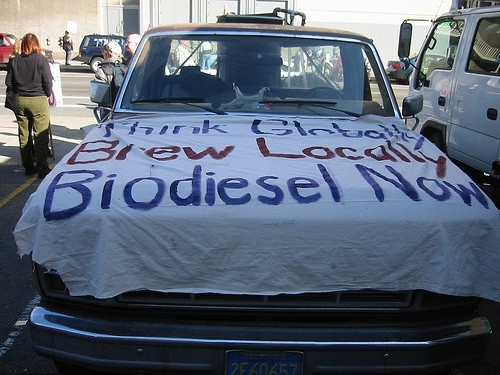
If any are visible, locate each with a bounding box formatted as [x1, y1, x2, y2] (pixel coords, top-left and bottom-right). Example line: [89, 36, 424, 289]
[5, 96, 11, 109]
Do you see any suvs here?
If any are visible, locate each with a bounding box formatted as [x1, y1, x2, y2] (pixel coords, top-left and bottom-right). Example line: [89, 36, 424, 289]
[77, 33, 132, 74]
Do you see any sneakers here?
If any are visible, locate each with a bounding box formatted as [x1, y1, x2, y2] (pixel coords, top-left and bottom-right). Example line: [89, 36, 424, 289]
[24, 163, 52, 179]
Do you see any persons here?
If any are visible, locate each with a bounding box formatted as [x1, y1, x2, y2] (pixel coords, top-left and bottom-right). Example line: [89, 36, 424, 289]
[63, 30, 74, 65]
[95, 41, 128, 121]
[124, 33, 142, 66]
[200, 41, 213, 70]
[5, 33, 53, 179]
[5, 38, 34, 119]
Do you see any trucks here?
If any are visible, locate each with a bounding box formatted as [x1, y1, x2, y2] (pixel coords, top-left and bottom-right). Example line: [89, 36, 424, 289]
[396, 6, 500, 184]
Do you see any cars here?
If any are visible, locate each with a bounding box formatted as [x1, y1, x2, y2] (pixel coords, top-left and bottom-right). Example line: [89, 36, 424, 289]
[14, 7, 500, 375]
[386, 53, 449, 86]
[0, 32, 53, 70]
